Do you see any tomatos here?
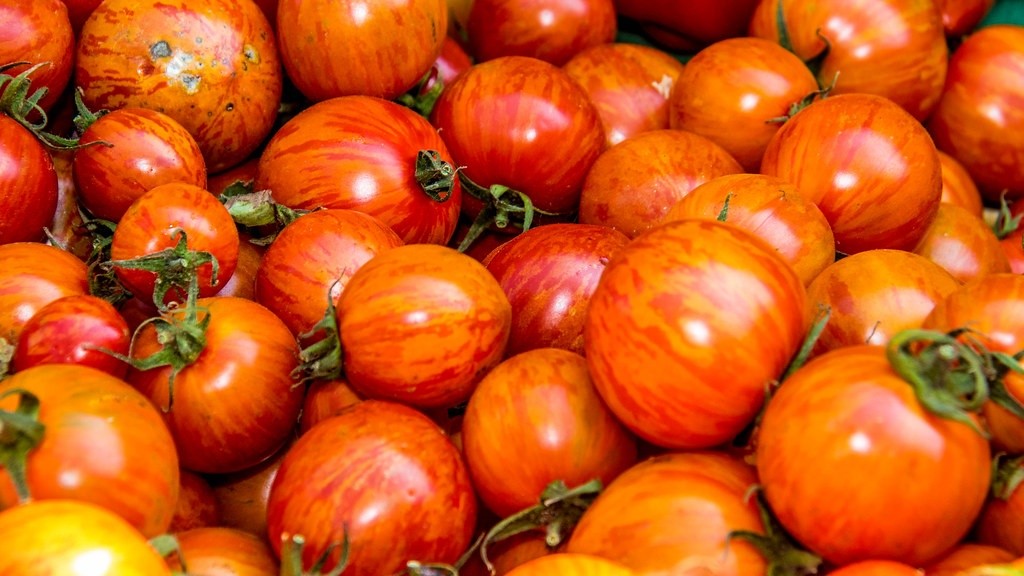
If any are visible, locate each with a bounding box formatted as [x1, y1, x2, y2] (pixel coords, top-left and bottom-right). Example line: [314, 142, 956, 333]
[0, 0, 1024, 576]
